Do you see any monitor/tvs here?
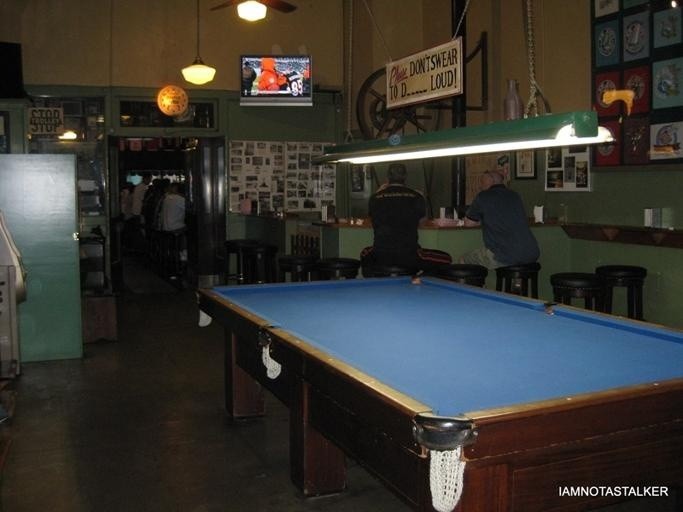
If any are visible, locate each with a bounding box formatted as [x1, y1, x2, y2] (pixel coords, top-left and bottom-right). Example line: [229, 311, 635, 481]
[239, 54, 313, 107]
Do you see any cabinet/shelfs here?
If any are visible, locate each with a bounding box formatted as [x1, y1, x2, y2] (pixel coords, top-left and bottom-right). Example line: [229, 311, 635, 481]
[36, 137, 110, 297]
[0, 146, 87, 366]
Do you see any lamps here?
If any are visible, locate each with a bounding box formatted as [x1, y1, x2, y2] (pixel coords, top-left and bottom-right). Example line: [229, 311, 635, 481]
[306, 1, 614, 168]
[235, 3, 269, 23]
[177, 0, 217, 85]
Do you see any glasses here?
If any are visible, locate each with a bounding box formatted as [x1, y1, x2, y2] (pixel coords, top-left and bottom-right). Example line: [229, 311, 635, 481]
[483, 169, 495, 184]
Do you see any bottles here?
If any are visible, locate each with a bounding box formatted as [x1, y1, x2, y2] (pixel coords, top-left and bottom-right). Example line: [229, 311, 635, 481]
[504, 78, 525, 120]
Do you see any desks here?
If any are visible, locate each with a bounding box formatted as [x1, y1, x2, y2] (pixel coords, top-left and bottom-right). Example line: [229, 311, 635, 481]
[193, 275, 680, 511]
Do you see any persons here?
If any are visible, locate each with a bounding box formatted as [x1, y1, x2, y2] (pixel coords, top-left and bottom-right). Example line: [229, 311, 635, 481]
[119, 172, 199, 281]
[242, 58, 310, 97]
[357, 163, 452, 277]
[458, 172, 540, 293]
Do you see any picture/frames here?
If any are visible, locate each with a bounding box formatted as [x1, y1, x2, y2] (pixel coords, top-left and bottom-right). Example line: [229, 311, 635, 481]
[588, 0, 682, 174]
[513, 145, 538, 182]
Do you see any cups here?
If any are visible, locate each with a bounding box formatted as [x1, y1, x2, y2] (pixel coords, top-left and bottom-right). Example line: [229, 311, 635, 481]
[642, 208, 653, 227]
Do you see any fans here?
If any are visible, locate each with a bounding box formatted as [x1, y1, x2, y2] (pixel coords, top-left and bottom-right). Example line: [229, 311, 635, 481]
[206, 0, 297, 17]
